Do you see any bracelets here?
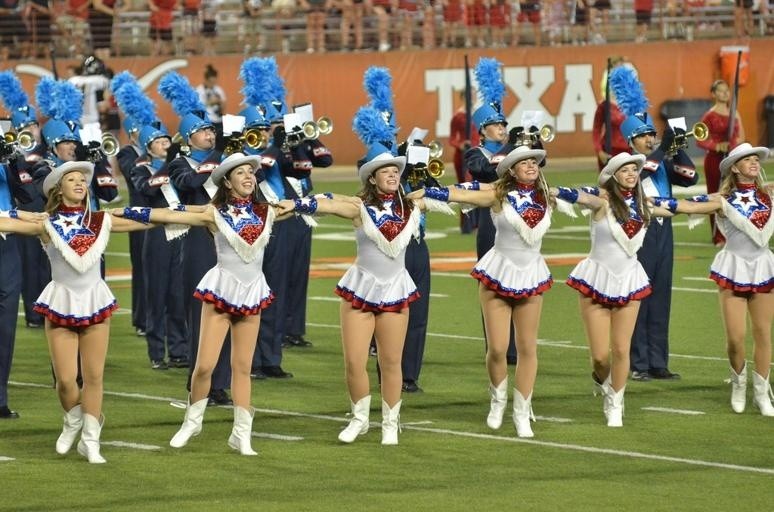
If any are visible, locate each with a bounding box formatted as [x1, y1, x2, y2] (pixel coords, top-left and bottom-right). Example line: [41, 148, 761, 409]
[454, 180, 480, 192]
[579, 184, 599, 197]
[651, 195, 677, 214]
[682, 192, 709, 202]
[422, 186, 450, 203]
[555, 186, 579, 206]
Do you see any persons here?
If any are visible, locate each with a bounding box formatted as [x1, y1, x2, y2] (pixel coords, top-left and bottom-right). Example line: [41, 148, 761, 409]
[193, 64, 233, 148]
[350, 142, 432, 393]
[66, 56, 123, 176]
[366, 110, 398, 357]
[3, 102, 338, 420]
[1, 0, 770, 62]
[450, 89, 479, 234]
[548, 151, 721, 430]
[697, 79, 747, 244]
[643, 143, 774, 420]
[460, 100, 555, 370]
[404, 144, 610, 441]
[267, 150, 497, 449]
[591, 93, 636, 173]
[104, 150, 362, 458]
[0, 160, 213, 466]
[605, 116, 710, 382]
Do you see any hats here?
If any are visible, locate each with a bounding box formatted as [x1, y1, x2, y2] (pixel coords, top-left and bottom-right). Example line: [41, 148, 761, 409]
[597, 151, 647, 188]
[718, 142, 769, 174]
[494, 145, 547, 178]
[355, 63, 399, 158]
[358, 151, 408, 186]
[472, 56, 509, 133]
[211, 152, 263, 185]
[42, 160, 95, 201]
[0, 55, 290, 151]
[608, 61, 657, 142]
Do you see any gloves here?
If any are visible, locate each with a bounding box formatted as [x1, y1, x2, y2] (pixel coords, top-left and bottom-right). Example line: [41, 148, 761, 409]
[507, 126, 524, 144]
[657, 127, 675, 151]
[675, 127, 687, 145]
[165, 142, 181, 164]
[89, 140, 103, 155]
[529, 126, 542, 147]
[75, 143, 88, 160]
[398, 141, 407, 156]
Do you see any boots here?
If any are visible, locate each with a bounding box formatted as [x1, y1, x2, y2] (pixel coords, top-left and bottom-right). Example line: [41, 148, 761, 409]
[603, 384, 627, 427]
[76, 413, 107, 464]
[381, 398, 403, 446]
[170, 394, 210, 448]
[55, 403, 83, 455]
[752, 368, 774, 417]
[512, 388, 535, 438]
[724, 359, 747, 413]
[227, 405, 258, 456]
[591, 370, 611, 396]
[338, 395, 371, 444]
[487, 374, 508, 431]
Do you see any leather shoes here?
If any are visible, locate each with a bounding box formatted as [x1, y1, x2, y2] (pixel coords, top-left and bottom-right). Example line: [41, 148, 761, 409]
[650, 368, 682, 379]
[280, 336, 292, 347]
[212, 390, 233, 405]
[150, 360, 168, 370]
[264, 364, 292, 378]
[0, 406, 20, 418]
[630, 369, 651, 381]
[251, 367, 266, 379]
[167, 358, 190, 368]
[401, 381, 424, 395]
[288, 335, 313, 347]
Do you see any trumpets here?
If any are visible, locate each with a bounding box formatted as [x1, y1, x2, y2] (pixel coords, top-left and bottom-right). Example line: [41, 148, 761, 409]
[2, 130, 38, 157]
[500, 125, 557, 146]
[286, 116, 333, 136]
[223, 128, 263, 156]
[85, 136, 120, 157]
[101, 131, 114, 140]
[172, 131, 184, 144]
[647, 122, 709, 152]
[3, 132, 17, 144]
[266, 121, 320, 147]
[407, 141, 444, 188]
